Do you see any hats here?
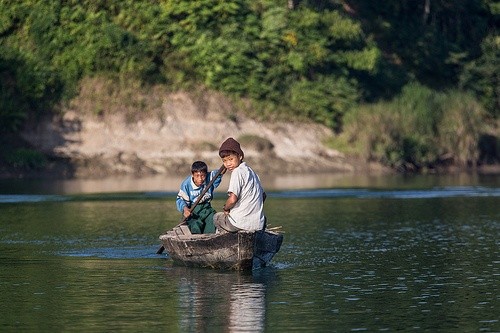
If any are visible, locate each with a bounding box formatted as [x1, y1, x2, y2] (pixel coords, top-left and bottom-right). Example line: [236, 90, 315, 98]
[219, 137, 245, 156]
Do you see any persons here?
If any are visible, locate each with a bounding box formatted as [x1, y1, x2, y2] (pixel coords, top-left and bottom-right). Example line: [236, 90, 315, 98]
[213, 138, 267, 234]
[176, 161, 227, 234]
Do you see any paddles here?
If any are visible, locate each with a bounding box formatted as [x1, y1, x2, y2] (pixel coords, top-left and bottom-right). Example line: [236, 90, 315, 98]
[156, 165, 226, 254]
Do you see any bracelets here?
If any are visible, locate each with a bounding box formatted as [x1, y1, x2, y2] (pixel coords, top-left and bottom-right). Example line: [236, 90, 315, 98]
[223, 208, 229, 211]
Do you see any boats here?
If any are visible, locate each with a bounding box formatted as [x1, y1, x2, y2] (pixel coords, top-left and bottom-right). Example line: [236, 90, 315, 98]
[158, 222, 284, 274]
